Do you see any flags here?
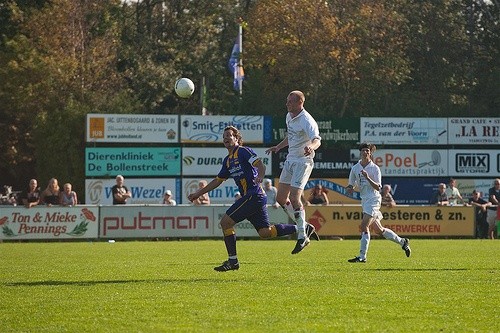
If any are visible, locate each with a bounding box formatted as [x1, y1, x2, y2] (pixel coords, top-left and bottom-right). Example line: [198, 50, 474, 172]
[228, 34, 245, 91]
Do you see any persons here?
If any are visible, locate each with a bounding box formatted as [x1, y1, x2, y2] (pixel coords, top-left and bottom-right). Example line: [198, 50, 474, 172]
[345, 143, 411, 263]
[263, 179, 280, 208]
[193, 180, 210, 204]
[445, 178, 468, 206]
[311, 183, 329, 205]
[489, 179, 500, 210]
[0, 178, 77, 207]
[468, 189, 492, 238]
[112, 175, 132, 205]
[188, 126, 319, 271]
[429, 183, 449, 206]
[380, 184, 395, 207]
[265, 90, 321, 254]
[159, 190, 176, 205]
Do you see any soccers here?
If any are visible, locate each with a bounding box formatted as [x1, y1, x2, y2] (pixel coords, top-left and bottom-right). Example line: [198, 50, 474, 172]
[174, 77, 195, 98]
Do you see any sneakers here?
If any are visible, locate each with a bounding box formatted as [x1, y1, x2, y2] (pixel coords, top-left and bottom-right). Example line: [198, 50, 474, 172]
[312, 232, 320, 241]
[214, 258, 239, 272]
[348, 256, 366, 263]
[291, 237, 310, 254]
[306, 223, 315, 237]
[402, 238, 411, 257]
